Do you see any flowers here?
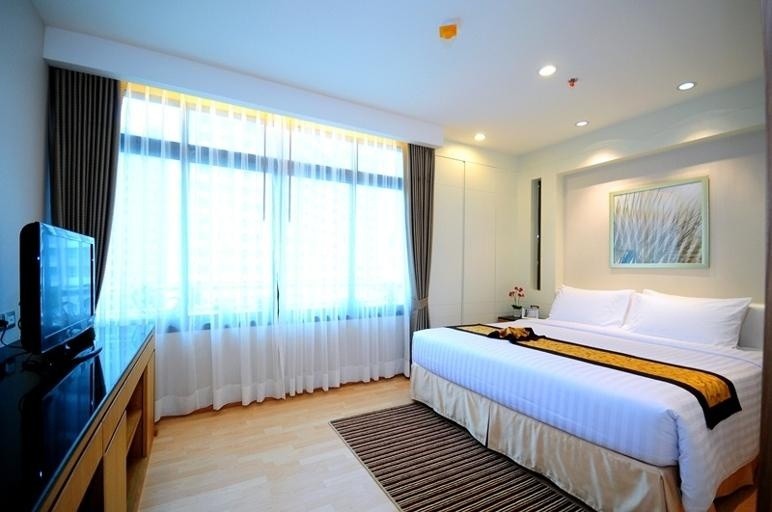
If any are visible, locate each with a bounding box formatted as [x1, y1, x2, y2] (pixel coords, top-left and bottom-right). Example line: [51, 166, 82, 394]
[507, 285, 524, 306]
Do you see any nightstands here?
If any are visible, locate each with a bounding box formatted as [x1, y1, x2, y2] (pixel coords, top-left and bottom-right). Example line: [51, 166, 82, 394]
[498, 315, 545, 322]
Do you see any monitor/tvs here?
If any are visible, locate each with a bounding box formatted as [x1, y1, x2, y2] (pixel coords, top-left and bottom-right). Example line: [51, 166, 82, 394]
[20, 354, 106, 512]
[20, 221, 102, 362]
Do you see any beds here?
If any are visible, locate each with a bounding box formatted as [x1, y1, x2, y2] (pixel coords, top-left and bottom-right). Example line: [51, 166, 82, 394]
[408, 285, 763, 509]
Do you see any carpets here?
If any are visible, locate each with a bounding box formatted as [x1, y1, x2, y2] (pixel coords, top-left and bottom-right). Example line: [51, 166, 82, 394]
[325, 399, 591, 509]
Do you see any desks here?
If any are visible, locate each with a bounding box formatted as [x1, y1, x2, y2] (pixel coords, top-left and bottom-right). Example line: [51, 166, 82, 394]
[0, 326, 158, 511]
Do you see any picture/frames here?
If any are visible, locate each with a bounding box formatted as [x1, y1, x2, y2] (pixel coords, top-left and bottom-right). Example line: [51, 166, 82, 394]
[608, 175, 709, 270]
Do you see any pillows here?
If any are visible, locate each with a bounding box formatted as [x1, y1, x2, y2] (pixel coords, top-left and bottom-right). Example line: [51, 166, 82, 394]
[543, 287, 632, 327]
[624, 292, 751, 347]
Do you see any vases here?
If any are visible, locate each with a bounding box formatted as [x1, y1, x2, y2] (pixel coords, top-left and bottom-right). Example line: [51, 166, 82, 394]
[511, 306, 523, 317]
